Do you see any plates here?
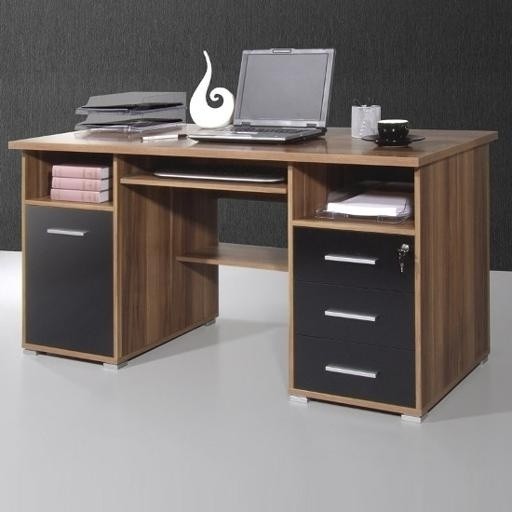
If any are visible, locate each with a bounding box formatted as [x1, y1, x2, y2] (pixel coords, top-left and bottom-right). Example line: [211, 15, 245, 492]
[362, 134, 427, 148]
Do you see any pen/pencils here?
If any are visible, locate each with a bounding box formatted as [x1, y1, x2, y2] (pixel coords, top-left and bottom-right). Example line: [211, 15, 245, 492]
[353, 97, 374, 107]
[141, 134, 188, 141]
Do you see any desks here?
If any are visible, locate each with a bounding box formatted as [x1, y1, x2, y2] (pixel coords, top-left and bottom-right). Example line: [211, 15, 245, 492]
[5, 123, 500, 424]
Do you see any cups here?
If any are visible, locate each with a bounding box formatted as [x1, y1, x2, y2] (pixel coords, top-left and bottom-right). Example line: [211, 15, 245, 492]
[377, 119, 409, 139]
[350, 105, 381, 140]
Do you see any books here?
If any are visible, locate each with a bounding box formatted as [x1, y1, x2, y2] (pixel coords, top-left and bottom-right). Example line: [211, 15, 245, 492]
[49, 164, 111, 205]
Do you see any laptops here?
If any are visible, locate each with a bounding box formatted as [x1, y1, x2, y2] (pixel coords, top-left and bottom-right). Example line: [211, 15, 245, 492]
[188, 48, 337, 145]
